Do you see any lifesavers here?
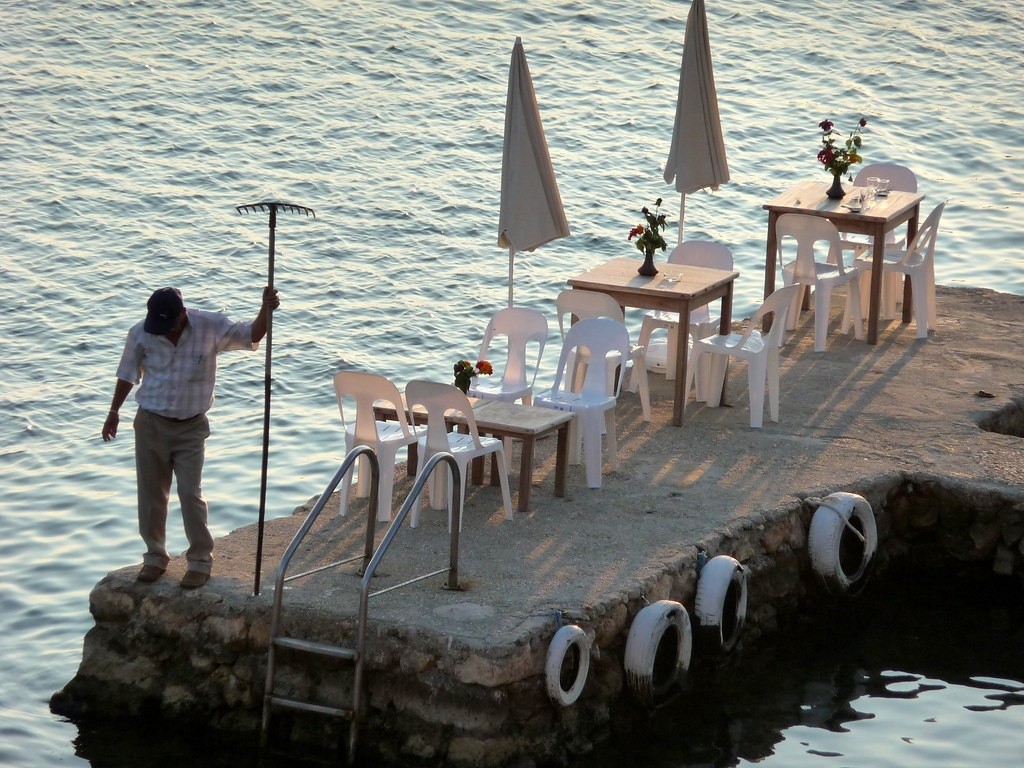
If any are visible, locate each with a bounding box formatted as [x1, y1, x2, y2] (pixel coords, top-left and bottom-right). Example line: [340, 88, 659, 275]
[543, 625, 590, 708]
[623, 599, 693, 710]
[693, 555, 747, 656]
[808, 491, 878, 603]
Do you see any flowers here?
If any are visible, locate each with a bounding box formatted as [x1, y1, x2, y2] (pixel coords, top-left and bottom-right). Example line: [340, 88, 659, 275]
[628, 198, 668, 253]
[454, 361, 492, 391]
[817, 117, 867, 176]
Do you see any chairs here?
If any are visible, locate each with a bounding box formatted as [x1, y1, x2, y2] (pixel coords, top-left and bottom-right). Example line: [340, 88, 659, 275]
[684, 283, 800, 428]
[776, 213, 864, 351]
[842, 201, 946, 340]
[534, 316, 629, 487]
[451, 308, 547, 475]
[557, 290, 651, 434]
[828, 163, 917, 319]
[334, 371, 427, 521]
[405, 380, 513, 533]
[629, 240, 733, 403]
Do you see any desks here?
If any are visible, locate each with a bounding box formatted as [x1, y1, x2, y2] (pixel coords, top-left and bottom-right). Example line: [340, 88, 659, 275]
[376, 393, 575, 510]
[761, 180, 925, 345]
[567, 259, 740, 427]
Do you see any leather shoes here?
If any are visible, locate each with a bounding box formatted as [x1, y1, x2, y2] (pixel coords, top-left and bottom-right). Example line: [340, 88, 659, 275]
[137, 565, 166, 584]
[180, 570, 209, 589]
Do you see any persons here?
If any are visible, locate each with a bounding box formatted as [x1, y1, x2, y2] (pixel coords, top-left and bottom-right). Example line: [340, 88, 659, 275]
[102, 286, 280, 589]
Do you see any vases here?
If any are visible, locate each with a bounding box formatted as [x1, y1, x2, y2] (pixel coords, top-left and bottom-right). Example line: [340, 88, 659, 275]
[455, 379, 467, 395]
[638, 247, 659, 276]
[826, 175, 846, 199]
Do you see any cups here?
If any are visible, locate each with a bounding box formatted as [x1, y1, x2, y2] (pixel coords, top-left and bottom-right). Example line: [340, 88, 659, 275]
[850, 177, 889, 211]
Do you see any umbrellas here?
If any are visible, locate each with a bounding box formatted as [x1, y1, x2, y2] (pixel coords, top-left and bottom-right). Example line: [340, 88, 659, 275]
[662, 0, 731, 243]
[498, 37, 571, 307]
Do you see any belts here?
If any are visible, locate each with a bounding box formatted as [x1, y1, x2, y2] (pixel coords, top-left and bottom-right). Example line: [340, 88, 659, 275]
[147, 410, 202, 423]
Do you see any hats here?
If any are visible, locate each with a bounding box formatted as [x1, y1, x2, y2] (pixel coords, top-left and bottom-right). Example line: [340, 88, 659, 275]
[145, 287, 182, 328]
